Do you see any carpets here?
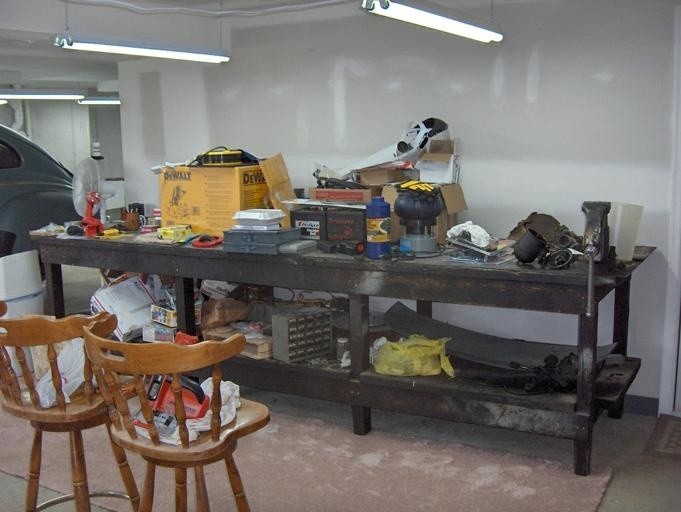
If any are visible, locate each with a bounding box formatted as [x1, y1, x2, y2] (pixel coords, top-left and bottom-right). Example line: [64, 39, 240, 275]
[641, 413, 681, 460]
[0, 392, 612, 512]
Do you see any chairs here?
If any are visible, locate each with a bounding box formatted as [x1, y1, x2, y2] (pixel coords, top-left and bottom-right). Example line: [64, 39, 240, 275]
[82, 313, 270, 512]
[0, 310, 140, 512]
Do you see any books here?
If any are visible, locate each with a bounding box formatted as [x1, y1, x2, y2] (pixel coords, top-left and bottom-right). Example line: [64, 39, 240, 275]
[445, 234, 517, 257]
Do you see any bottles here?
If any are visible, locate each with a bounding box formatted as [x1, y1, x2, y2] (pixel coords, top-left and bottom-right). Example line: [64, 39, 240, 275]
[335, 337, 350, 362]
[366, 195, 392, 261]
[147, 373, 163, 401]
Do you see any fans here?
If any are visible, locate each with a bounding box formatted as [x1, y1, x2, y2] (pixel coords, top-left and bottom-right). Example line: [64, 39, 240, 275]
[71, 158, 118, 230]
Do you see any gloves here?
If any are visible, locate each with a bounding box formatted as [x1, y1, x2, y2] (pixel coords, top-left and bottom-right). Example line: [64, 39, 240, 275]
[395, 179, 439, 202]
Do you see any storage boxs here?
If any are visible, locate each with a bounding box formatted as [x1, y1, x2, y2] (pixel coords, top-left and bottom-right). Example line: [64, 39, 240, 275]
[290, 138, 468, 245]
[161, 164, 271, 239]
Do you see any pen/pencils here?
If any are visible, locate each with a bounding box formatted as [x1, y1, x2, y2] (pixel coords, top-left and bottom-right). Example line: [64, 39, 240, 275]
[126, 202, 138, 213]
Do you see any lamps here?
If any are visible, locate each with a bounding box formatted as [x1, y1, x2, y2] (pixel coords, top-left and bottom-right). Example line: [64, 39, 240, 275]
[50, 0, 232, 65]
[359, 0, 504, 44]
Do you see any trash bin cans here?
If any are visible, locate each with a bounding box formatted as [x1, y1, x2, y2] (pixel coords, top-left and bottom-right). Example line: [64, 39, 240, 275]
[0, 250, 45, 378]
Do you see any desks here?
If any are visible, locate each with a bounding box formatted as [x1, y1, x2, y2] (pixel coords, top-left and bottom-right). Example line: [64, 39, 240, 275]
[29, 201, 656, 474]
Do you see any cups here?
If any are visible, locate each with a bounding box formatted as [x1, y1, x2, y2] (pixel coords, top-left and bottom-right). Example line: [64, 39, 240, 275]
[122, 213, 140, 230]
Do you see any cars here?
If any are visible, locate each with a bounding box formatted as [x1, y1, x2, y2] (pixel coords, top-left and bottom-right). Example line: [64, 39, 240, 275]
[0, 124, 100, 258]
